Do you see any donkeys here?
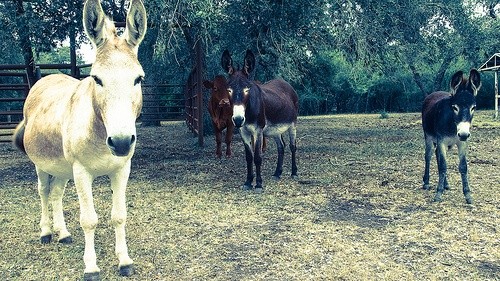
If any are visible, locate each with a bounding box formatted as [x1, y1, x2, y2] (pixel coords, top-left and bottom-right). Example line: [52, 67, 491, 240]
[203, 76, 235, 160]
[221, 48, 299, 193]
[421, 68, 482, 205]
[14, 0, 147, 281]
